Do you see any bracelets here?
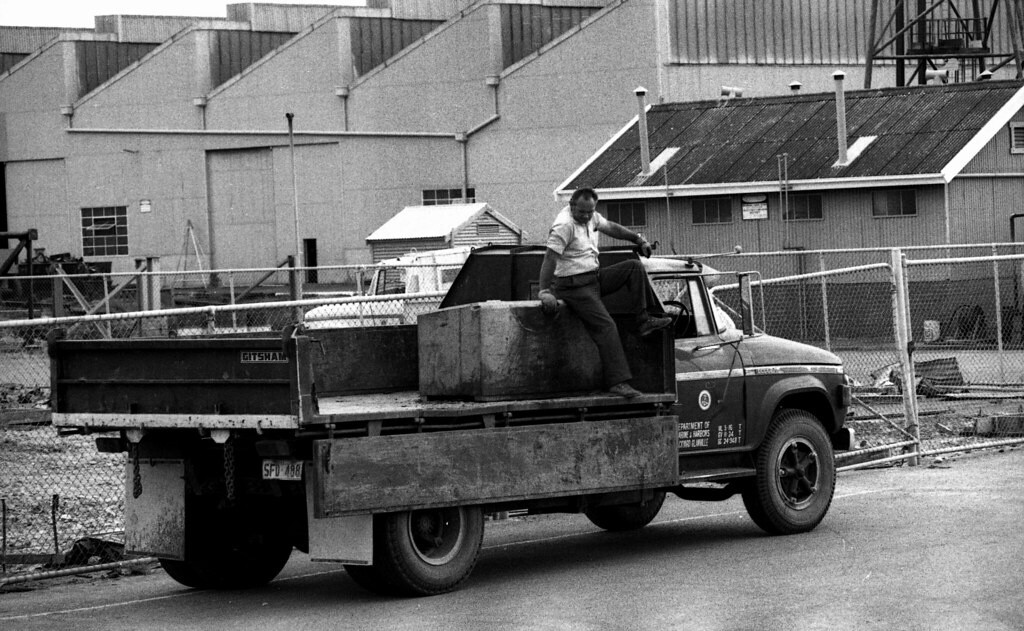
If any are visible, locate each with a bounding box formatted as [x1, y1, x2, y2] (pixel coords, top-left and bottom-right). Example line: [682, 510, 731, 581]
[639, 240, 647, 247]
[538, 289, 551, 298]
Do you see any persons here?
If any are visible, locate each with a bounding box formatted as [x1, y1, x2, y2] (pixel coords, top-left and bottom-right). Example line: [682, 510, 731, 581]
[538, 187, 672, 397]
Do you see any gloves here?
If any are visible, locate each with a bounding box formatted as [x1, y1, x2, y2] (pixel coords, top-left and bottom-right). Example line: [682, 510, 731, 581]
[539, 289, 559, 312]
[636, 237, 652, 258]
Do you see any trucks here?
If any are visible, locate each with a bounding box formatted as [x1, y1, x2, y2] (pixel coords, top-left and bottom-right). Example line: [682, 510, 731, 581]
[45, 241, 862, 597]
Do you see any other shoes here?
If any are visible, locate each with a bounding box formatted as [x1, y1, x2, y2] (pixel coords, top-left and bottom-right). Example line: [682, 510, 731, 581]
[639, 317, 672, 337]
[609, 384, 638, 398]
